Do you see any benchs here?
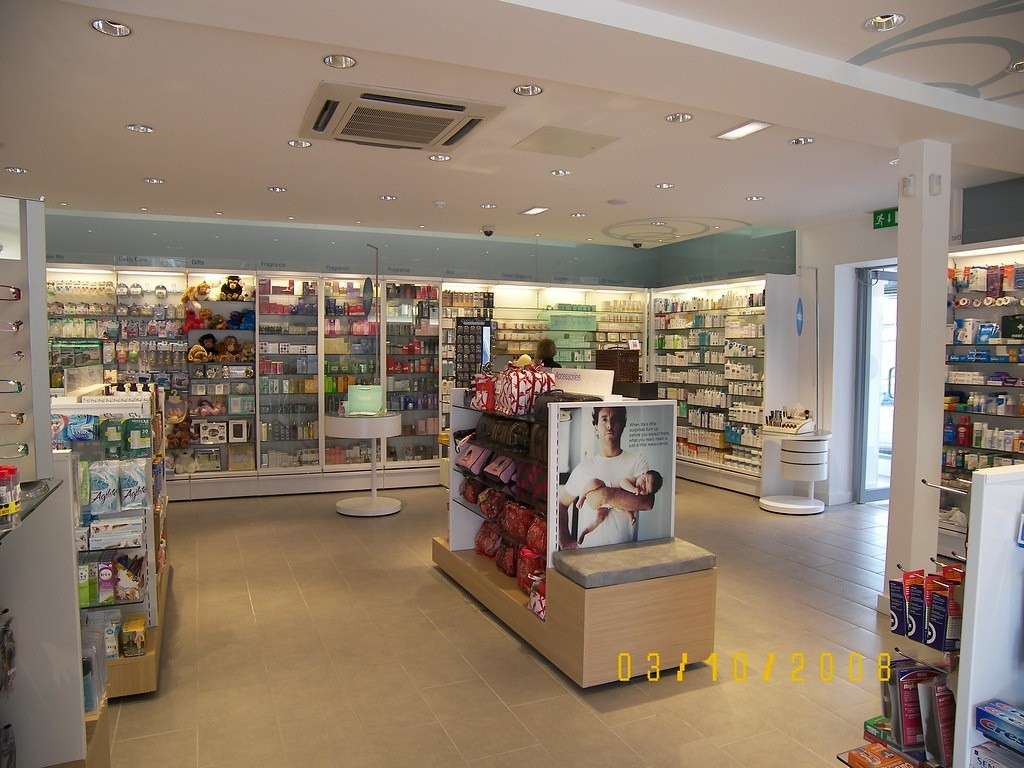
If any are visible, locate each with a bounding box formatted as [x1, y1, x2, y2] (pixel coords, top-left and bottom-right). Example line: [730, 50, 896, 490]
[547, 537, 718, 689]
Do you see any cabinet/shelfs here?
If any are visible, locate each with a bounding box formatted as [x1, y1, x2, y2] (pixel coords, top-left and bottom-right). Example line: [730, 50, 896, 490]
[46, 265, 797, 502]
[937, 234, 1024, 562]
[57, 382, 168, 699]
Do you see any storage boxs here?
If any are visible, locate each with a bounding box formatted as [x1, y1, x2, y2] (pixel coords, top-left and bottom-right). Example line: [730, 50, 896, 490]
[86, 552, 101, 603]
[121, 612, 146, 657]
[74, 527, 89, 541]
[89, 517, 145, 537]
[970, 697, 1024, 768]
[99, 549, 116, 605]
[90, 535, 142, 550]
[885, 562, 964, 652]
[848, 715, 916, 768]
[78, 563, 91, 609]
[74, 542, 89, 552]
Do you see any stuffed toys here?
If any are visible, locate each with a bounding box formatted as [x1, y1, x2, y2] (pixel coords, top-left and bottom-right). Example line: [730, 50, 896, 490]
[164, 276, 257, 474]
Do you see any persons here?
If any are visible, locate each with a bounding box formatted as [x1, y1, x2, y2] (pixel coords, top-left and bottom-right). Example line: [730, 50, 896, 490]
[558, 405, 663, 549]
[535, 338, 563, 369]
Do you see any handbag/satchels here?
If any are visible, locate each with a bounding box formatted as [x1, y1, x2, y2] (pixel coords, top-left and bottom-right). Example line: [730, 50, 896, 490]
[484, 455, 518, 484]
[534, 386, 604, 428]
[472, 374, 495, 412]
[494, 363, 556, 415]
[456, 443, 493, 476]
[476, 413, 548, 464]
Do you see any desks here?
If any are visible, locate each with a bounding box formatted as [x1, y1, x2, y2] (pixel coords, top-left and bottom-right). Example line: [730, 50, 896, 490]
[324, 412, 401, 518]
[755, 430, 834, 515]
